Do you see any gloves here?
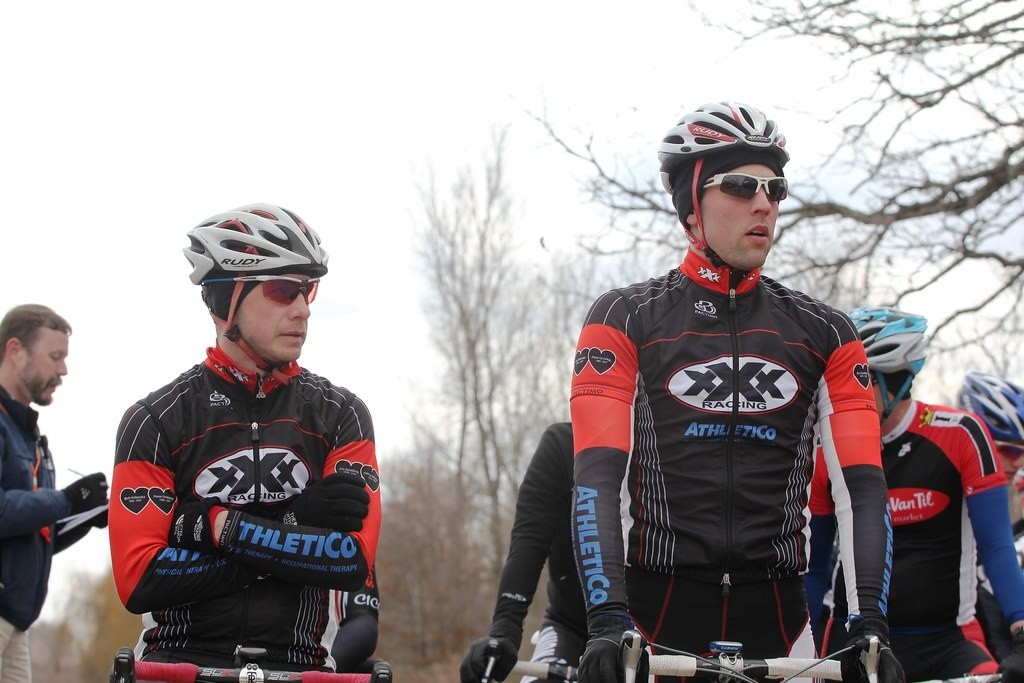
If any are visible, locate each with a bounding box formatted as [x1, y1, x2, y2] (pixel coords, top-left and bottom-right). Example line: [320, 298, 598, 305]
[460, 635, 518, 683]
[169, 493, 223, 553]
[62, 472, 109, 529]
[996, 626, 1024, 683]
[575, 612, 649, 683]
[840, 617, 905, 683]
[275, 472, 371, 531]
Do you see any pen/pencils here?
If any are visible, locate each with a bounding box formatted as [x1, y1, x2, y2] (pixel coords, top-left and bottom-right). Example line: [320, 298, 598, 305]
[68, 467, 86, 477]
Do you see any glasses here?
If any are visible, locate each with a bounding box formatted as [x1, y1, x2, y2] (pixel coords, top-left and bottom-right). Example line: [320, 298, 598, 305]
[258, 280, 320, 305]
[703, 174, 788, 201]
[995, 441, 1023, 460]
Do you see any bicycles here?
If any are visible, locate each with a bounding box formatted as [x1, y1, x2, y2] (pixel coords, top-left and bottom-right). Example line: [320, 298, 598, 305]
[105, 640, 1019, 682]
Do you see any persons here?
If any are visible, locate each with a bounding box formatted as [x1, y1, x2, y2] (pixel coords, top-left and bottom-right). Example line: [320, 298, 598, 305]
[320, 563, 381, 672]
[108, 220, 381, 683]
[569, 114, 907, 683]
[460, 422, 588, 683]
[951, 376, 1024, 544]
[811, 310, 1023, 683]
[2, 305, 107, 683]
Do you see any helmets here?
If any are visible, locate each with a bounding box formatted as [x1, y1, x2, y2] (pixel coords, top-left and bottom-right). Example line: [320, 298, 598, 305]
[658, 102, 791, 195]
[182, 204, 330, 286]
[956, 371, 1024, 457]
[843, 307, 929, 374]
